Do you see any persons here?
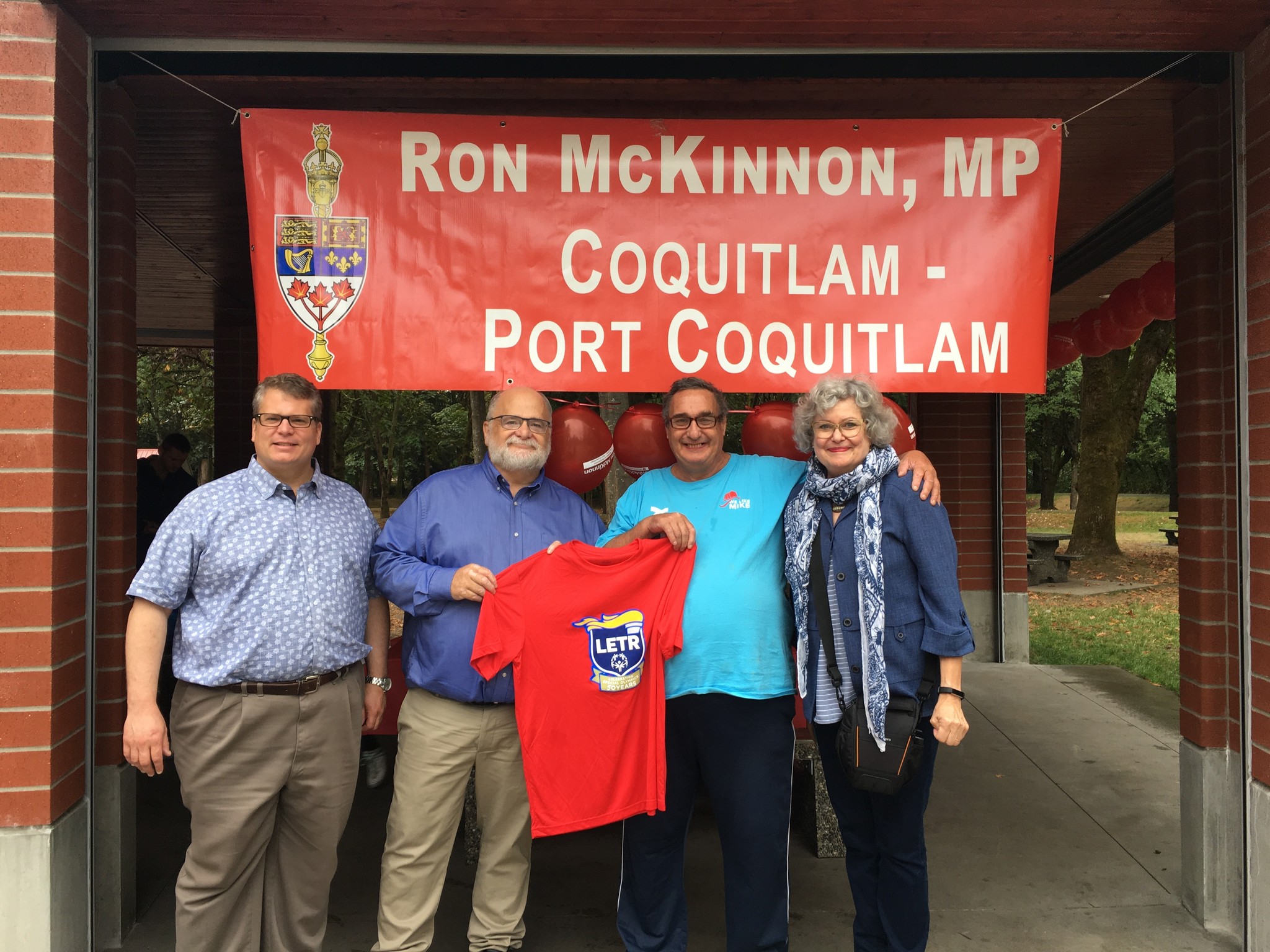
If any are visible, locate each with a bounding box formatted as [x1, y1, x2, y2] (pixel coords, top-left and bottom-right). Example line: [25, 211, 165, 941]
[784, 377, 978, 950]
[133, 429, 202, 583]
[368, 385, 606, 952]
[120, 371, 396, 952]
[592, 377, 945, 950]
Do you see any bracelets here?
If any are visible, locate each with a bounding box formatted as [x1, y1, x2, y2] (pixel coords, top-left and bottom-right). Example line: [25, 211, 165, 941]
[937, 686, 965, 701]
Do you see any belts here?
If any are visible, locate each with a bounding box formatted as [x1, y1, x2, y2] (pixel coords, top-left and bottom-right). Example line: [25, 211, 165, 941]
[219, 662, 356, 696]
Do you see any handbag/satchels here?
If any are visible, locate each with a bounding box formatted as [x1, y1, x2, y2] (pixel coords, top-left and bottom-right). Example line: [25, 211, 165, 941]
[836, 692, 926, 796]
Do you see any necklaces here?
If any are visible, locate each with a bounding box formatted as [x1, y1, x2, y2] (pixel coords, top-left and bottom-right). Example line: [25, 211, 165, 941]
[831, 502, 846, 513]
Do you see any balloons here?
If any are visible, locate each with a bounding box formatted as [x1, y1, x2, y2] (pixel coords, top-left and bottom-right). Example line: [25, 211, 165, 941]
[869, 394, 917, 457]
[606, 403, 676, 479]
[738, 399, 817, 466]
[537, 399, 616, 495]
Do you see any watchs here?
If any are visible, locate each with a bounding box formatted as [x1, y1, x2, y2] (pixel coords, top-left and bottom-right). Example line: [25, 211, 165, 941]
[362, 675, 393, 693]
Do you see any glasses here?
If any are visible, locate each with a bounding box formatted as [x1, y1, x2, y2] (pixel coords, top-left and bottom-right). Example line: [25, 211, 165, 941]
[253, 413, 319, 428]
[488, 415, 551, 434]
[665, 413, 723, 430]
[811, 419, 866, 440]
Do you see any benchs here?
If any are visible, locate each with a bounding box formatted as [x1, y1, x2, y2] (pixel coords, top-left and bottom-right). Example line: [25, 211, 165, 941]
[1027, 553, 1086, 583]
[1026, 554, 1045, 587]
[1159, 528, 1178, 546]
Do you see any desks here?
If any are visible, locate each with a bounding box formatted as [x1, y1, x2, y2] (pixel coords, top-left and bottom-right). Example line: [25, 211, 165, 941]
[1169, 516, 1179, 527]
[1025, 532, 1074, 587]
